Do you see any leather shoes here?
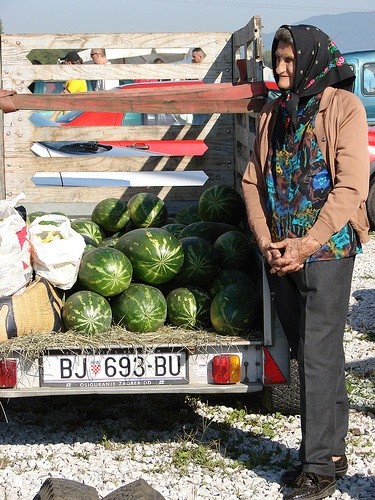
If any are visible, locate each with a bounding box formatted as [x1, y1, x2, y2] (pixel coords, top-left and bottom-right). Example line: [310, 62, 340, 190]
[294, 454, 348, 478]
[282, 471, 336, 500]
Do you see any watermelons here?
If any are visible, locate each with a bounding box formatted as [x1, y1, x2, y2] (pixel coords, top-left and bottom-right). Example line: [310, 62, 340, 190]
[28, 183, 260, 336]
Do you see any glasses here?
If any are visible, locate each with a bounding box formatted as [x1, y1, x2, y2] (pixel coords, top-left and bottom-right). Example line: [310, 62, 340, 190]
[90, 53, 96, 58]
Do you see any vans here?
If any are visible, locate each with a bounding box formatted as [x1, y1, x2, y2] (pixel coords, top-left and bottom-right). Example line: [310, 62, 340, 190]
[341, 49, 375, 230]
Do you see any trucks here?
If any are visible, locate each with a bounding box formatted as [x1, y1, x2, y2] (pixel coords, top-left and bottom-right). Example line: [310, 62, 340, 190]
[0, 14, 305, 416]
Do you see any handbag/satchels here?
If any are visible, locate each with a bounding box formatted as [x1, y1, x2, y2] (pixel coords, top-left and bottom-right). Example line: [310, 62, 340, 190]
[28, 212, 86, 290]
[0, 191, 33, 298]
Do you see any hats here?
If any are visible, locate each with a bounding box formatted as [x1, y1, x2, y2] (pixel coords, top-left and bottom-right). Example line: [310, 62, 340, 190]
[60, 52, 81, 61]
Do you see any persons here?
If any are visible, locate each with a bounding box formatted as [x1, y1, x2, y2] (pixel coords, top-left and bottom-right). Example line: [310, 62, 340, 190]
[241, 24, 371, 500]
[30, 47, 208, 126]
